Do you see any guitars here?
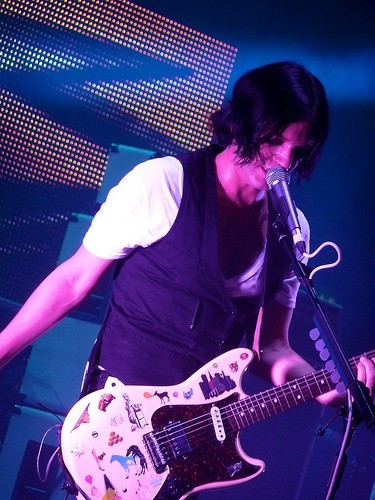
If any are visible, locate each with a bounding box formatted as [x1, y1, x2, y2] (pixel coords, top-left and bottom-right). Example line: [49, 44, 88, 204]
[56, 346, 375, 500]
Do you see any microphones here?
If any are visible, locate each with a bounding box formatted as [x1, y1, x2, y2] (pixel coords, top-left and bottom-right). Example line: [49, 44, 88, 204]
[265, 166, 308, 255]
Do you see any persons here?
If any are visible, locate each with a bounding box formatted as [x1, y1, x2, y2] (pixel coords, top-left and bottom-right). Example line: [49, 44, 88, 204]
[0, 62, 375, 500]
